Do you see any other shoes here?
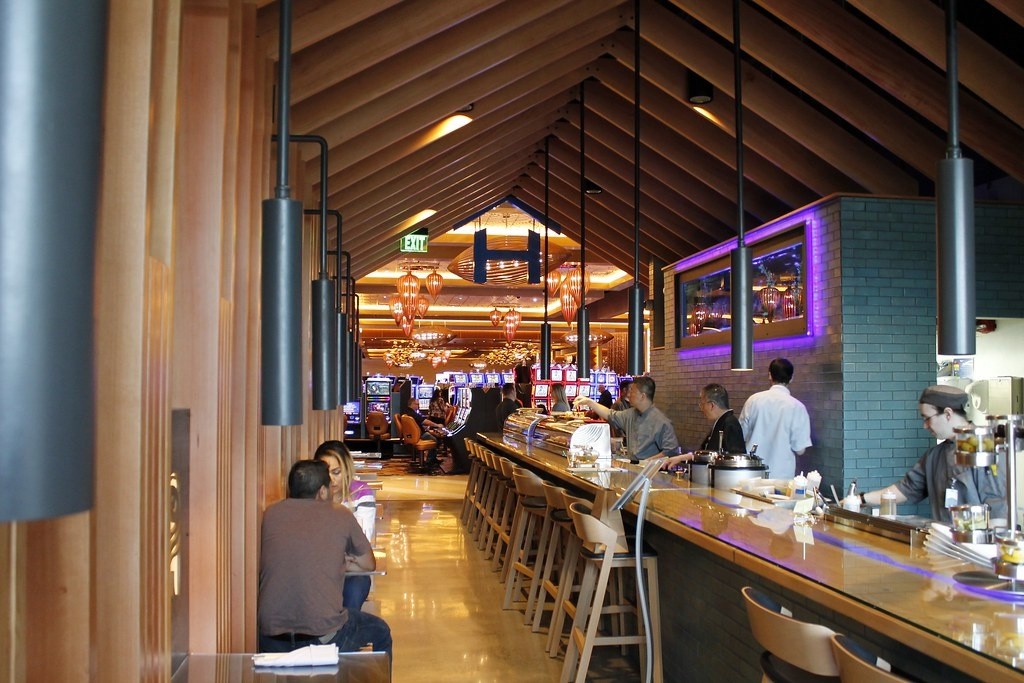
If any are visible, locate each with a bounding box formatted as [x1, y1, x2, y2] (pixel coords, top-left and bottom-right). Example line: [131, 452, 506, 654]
[436, 459, 444, 464]
[426, 459, 437, 466]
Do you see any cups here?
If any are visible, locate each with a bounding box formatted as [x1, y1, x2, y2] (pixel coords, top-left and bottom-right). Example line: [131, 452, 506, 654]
[567, 451, 631, 469]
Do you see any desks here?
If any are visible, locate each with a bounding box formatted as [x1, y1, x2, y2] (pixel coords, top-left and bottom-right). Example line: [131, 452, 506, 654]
[169, 650, 391, 683]
[345, 449, 388, 578]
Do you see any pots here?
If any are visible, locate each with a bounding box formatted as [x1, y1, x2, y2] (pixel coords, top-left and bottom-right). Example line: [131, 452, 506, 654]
[690, 449, 769, 494]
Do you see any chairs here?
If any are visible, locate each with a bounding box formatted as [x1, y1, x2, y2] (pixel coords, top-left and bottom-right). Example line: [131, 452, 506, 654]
[366, 411, 390, 459]
[394, 413, 438, 475]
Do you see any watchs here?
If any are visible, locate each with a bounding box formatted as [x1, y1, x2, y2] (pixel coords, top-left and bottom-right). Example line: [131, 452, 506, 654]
[858, 492, 866, 504]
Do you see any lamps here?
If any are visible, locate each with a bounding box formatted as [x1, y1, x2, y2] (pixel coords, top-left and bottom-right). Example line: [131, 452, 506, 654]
[385, 258, 443, 336]
[264, 132, 363, 413]
[540, 138, 550, 379]
[489, 302, 524, 344]
[576, 80, 590, 379]
[935, 0, 977, 355]
[260, 0, 303, 426]
[0, 1, 92, 523]
[626, 2, 645, 377]
[729, 1, 756, 372]
[545, 261, 590, 327]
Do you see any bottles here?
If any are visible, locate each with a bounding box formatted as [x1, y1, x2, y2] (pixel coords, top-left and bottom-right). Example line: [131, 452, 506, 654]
[786, 469, 822, 499]
[842, 494, 861, 513]
[879, 490, 896, 521]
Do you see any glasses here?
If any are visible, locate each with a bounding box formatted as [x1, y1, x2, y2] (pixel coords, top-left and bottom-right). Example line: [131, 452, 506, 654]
[922, 411, 943, 424]
[699, 400, 715, 408]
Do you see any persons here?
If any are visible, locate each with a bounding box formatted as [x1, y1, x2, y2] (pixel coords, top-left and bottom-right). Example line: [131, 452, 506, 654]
[261, 459, 393, 683]
[660, 382, 748, 470]
[573, 376, 679, 460]
[739, 357, 812, 481]
[839, 386, 1007, 531]
[315, 439, 376, 610]
[405, 380, 632, 475]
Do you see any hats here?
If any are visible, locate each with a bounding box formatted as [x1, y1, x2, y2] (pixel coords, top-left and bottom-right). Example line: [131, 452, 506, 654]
[919, 385, 969, 409]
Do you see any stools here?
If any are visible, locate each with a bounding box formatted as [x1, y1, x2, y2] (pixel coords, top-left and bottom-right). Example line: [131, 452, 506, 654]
[739, 582, 912, 683]
[459, 436, 664, 683]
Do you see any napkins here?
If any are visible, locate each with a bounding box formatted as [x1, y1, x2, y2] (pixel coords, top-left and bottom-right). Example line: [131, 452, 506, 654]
[253, 643, 338, 666]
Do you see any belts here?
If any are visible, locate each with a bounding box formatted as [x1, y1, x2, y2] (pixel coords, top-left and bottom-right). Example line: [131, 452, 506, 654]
[271, 633, 318, 642]
